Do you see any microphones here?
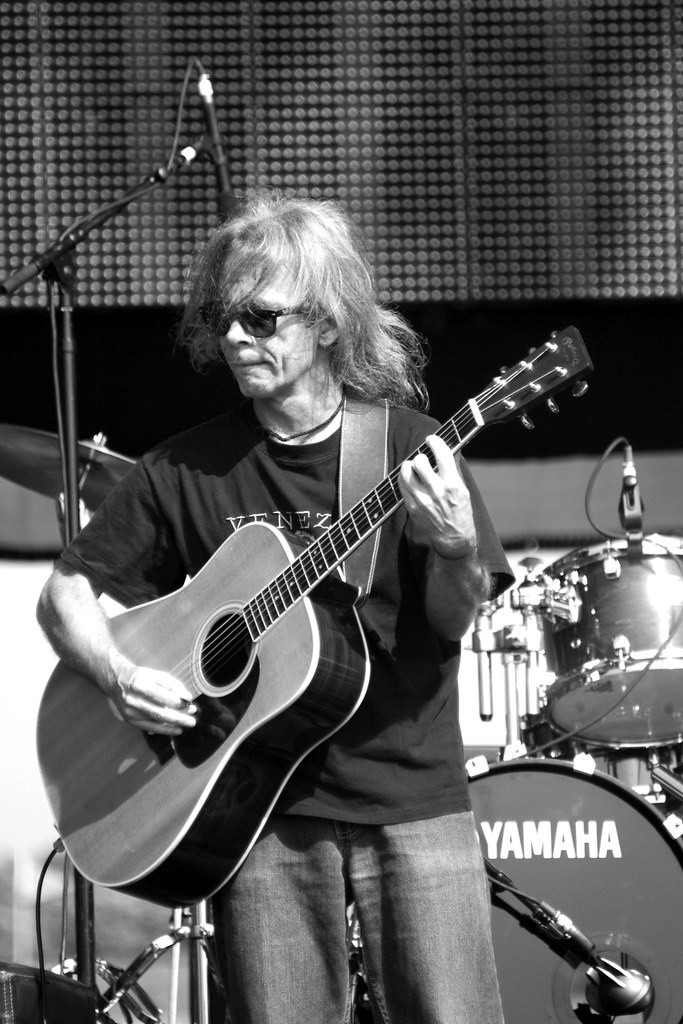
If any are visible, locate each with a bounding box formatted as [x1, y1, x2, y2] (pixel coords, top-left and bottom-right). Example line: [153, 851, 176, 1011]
[196, 77, 239, 223]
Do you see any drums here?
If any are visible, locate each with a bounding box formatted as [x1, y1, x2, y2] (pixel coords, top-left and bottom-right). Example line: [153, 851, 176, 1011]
[460, 758, 683, 1024]
[537, 535, 683, 749]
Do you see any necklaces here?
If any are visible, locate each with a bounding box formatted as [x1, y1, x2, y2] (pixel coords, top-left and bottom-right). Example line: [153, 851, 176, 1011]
[259, 395, 346, 442]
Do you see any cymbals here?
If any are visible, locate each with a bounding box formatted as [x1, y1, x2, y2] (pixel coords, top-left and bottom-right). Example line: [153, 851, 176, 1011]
[0, 423, 140, 506]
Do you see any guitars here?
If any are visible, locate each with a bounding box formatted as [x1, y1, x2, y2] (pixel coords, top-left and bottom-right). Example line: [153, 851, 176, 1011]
[36, 324, 594, 906]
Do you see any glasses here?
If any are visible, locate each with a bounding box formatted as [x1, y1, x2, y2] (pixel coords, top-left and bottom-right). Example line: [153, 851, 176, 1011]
[196, 302, 306, 338]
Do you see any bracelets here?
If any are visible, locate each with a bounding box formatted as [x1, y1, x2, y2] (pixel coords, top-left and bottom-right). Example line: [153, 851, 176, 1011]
[432, 531, 480, 561]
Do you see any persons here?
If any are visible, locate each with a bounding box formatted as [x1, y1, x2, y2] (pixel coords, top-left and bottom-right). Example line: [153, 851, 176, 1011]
[36, 194, 515, 1024]
